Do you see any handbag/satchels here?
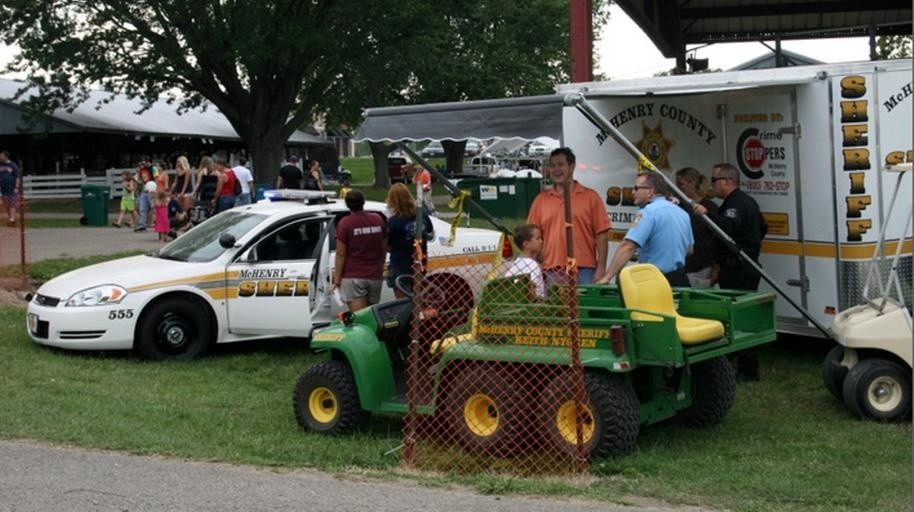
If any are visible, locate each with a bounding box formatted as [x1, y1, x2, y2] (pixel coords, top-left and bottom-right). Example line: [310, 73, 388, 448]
[305, 179, 317, 188]
[234, 179, 241, 197]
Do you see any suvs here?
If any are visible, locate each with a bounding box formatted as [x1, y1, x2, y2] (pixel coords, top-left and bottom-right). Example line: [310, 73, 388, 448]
[386, 152, 415, 185]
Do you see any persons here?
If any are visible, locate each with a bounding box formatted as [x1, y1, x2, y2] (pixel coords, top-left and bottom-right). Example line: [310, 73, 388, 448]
[505, 223, 546, 303]
[412, 165, 431, 187]
[673, 167, 722, 291]
[524, 146, 613, 286]
[593, 170, 695, 288]
[0, 151, 22, 228]
[278, 157, 323, 190]
[690, 163, 767, 291]
[112, 156, 256, 243]
[383, 182, 433, 300]
[324, 191, 390, 314]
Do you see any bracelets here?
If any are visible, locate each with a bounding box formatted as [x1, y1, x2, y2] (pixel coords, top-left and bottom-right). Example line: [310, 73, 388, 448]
[334, 281, 341, 288]
[14, 186, 20, 190]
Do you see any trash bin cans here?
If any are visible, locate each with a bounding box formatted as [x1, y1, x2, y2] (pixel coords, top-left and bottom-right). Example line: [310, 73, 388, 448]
[80, 184, 111, 226]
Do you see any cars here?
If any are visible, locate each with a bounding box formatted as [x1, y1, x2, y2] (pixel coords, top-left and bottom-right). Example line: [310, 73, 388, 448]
[465, 140, 480, 157]
[527, 140, 552, 157]
[24, 187, 512, 361]
[421, 141, 447, 157]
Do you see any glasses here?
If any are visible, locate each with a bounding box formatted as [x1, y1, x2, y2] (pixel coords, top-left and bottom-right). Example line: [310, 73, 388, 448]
[711, 177, 726, 182]
[636, 185, 650, 190]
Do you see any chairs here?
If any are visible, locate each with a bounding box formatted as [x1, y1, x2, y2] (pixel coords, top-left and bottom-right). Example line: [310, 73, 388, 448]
[277, 223, 319, 259]
[428, 306, 478, 356]
[619, 262, 725, 349]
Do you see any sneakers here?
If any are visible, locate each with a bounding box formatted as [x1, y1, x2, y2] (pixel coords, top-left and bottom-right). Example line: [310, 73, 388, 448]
[113, 221, 146, 232]
